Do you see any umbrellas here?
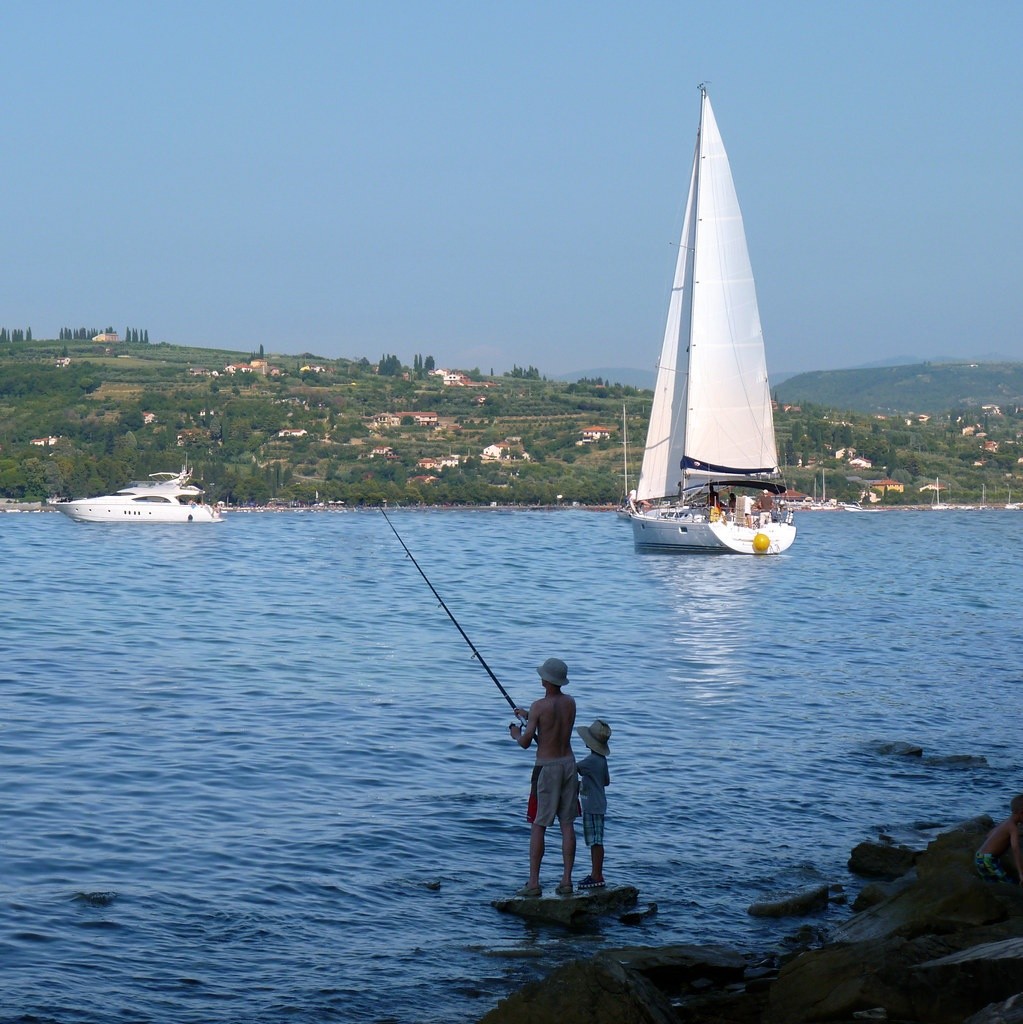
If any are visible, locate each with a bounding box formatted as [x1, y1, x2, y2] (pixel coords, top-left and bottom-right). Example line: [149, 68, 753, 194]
[335, 500, 344, 505]
[328, 500, 334, 505]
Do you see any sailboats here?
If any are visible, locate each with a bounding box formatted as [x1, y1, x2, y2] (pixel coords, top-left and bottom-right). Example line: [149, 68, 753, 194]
[620, 83, 800, 555]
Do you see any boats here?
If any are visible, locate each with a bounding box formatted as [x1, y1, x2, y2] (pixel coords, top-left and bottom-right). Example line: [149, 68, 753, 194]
[48, 465, 226, 525]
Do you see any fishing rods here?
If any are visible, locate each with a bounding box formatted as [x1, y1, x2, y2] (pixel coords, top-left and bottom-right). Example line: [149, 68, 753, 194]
[374, 503, 545, 752]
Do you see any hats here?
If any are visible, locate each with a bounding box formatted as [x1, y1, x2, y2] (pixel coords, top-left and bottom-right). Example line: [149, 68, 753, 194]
[577, 720, 611, 756]
[537, 658, 569, 685]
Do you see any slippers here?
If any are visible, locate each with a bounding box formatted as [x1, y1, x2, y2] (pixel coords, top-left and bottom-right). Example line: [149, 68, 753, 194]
[577, 875, 605, 888]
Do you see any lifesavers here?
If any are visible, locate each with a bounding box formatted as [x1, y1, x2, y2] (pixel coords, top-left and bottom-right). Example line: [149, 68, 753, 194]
[637, 500, 648, 512]
[710, 507, 719, 522]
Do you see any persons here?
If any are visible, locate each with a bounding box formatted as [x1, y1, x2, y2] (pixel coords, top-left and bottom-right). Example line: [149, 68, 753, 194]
[975, 794, 1023, 894]
[756, 489, 775, 524]
[728, 493, 737, 507]
[574, 720, 611, 888]
[188, 498, 221, 518]
[741, 490, 754, 529]
[510, 658, 582, 897]
[706, 485, 719, 506]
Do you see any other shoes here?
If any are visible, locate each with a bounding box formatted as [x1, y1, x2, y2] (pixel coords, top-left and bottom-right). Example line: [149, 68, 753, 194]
[516, 882, 542, 896]
[555, 883, 573, 893]
